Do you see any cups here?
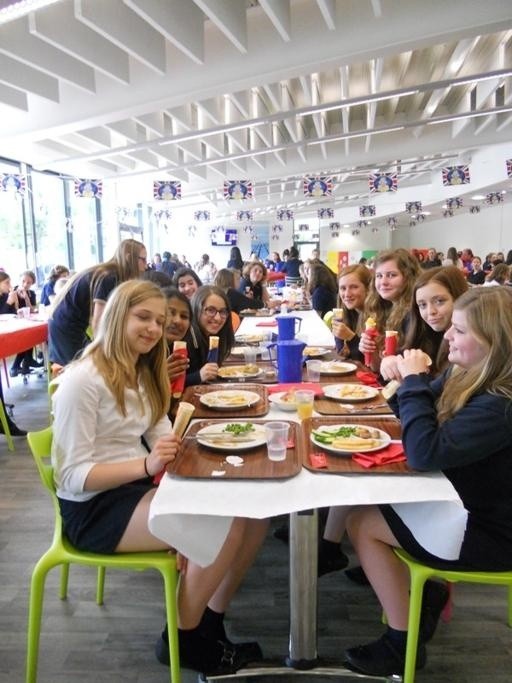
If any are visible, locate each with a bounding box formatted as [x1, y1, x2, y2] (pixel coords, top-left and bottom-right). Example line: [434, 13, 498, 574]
[242, 302, 324, 463]
[17, 303, 52, 322]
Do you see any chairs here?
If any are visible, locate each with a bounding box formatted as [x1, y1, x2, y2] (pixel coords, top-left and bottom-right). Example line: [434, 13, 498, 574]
[381, 547, 512, 683]
[25, 374, 181, 683]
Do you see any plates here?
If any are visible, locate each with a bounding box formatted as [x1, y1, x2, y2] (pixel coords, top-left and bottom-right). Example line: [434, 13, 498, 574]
[307, 361, 358, 375]
[217, 365, 263, 378]
[230, 346, 269, 355]
[308, 424, 392, 456]
[195, 422, 274, 451]
[321, 384, 380, 402]
[233, 334, 263, 342]
[199, 390, 260, 411]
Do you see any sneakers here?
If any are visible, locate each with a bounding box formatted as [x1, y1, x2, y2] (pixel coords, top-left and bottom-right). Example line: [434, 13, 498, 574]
[198, 625, 263, 666]
[9, 360, 41, 377]
[318, 550, 350, 577]
[0, 421, 27, 436]
[156, 631, 247, 676]
[344, 633, 428, 675]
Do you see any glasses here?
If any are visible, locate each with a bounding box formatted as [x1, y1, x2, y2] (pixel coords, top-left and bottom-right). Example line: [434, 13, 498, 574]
[201, 305, 230, 319]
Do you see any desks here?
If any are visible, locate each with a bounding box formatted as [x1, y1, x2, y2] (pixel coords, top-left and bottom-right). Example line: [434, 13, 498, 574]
[1, 303, 48, 390]
[148, 286, 468, 682]
